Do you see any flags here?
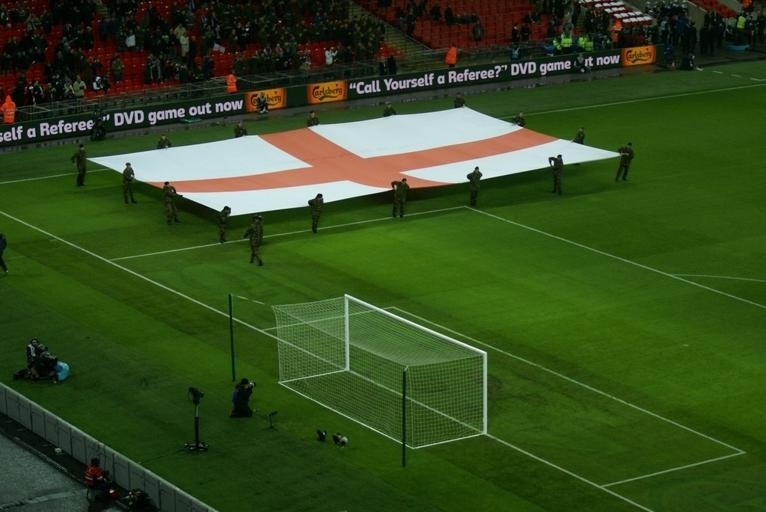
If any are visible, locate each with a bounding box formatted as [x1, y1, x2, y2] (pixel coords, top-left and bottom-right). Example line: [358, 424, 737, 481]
[87, 103, 622, 219]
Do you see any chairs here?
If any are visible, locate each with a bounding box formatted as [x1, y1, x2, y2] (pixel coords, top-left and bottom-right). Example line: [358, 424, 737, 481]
[689, 0, 735, 17]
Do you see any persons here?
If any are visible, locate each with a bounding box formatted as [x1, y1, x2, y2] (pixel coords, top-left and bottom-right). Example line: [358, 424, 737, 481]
[217, 206, 231, 244]
[229, 377, 256, 416]
[384, 102, 397, 117]
[84, 458, 107, 491]
[157, 133, 171, 148]
[1, 2, 484, 125]
[570, 127, 585, 146]
[454, 93, 466, 108]
[466, 166, 482, 207]
[89, 110, 106, 142]
[13, 338, 71, 383]
[121, 163, 137, 204]
[307, 193, 323, 234]
[615, 143, 635, 183]
[391, 178, 410, 218]
[548, 154, 563, 195]
[307, 112, 318, 127]
[242, 215, 263, 267]
[0, 234, 10, 273]
[161, 182, 182, 226]
[70, 144, 87, 187]
[510, 1, 765, 71]
[234, 120, 247, 139]
[512, 111, 526, 129]
